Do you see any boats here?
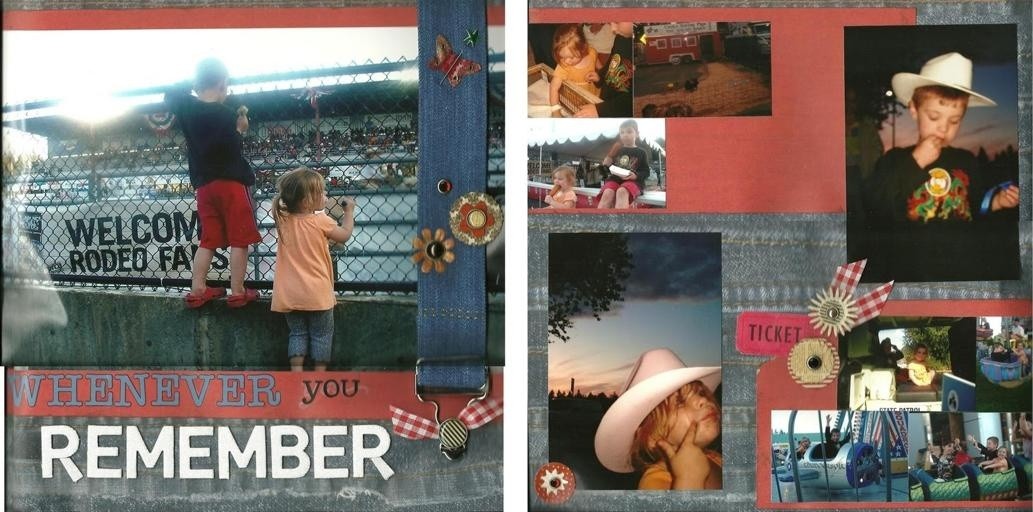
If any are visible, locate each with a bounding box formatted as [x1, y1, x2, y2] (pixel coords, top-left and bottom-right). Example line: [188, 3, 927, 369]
[528, 133, 666, 208]
[909, 455, 1031, 501]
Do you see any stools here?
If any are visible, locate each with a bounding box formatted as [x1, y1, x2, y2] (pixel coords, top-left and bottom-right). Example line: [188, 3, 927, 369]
[932, 383, 943, 400]
[895, 380, 936, 401]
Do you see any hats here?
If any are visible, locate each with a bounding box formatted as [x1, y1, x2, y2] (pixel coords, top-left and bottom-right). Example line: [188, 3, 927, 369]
[890, 52, 998, 107]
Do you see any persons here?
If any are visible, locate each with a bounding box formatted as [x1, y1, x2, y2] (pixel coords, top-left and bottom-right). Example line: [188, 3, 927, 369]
[550, 25, 604, 118]
[994, 318, 1029, 368]
[164, 58, 263, 309]
[825, 415, 850, 458]
[542, 164, 578, 210]
[871, 51, 1019, 281]
[926, 412, 1033, 484]
[800, 437, 810, 459]
[601, 22, 633, 117]
[908, 344, 935, 386]
[270, 168, 354, 371]
[594, 120, 650, 209]
[880, 337, 904, 368]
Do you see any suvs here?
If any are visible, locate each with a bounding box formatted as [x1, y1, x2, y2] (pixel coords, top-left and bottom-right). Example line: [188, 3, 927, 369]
[724, 23, 770, 68]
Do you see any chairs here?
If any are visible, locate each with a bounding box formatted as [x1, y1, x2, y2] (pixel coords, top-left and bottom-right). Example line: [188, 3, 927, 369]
[22, 129, 505, 202]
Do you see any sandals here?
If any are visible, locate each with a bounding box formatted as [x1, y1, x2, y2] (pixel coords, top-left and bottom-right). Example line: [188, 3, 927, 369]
[186, 287, 226, 309]
[226, 288, 260, 308]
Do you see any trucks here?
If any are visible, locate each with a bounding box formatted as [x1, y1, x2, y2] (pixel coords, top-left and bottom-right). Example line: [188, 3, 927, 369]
[643, 22, 722, 66]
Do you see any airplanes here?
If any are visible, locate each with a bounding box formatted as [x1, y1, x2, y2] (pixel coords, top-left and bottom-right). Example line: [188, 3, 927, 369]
[771, 438, 881, 493]
